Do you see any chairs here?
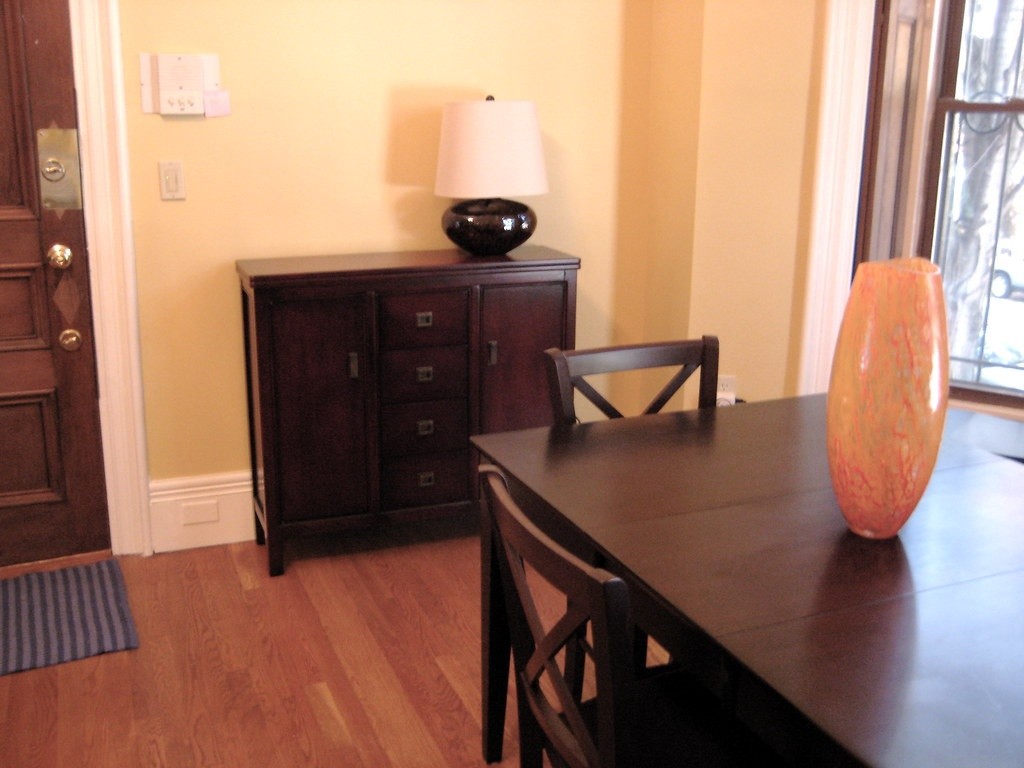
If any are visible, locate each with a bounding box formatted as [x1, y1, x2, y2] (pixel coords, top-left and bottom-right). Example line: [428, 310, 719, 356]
[540, 334, 719, 424]
[479, 457, 848, 768]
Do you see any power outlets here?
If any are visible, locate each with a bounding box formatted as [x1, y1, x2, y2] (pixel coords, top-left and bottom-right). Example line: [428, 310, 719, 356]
[716, 375, 737, 407]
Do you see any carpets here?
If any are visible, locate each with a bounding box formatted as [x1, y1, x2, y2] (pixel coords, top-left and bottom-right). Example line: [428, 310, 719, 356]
[0, 551, 143, 678]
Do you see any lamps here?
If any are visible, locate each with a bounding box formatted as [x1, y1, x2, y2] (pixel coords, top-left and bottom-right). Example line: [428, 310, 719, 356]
[432, 90, 555, 257]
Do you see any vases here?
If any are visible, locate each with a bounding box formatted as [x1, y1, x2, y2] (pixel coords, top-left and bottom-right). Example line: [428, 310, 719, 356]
[824, 254, 954, 543]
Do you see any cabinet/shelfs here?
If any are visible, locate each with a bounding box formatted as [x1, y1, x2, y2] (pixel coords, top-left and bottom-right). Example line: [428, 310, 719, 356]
[235, 240, 581, 580]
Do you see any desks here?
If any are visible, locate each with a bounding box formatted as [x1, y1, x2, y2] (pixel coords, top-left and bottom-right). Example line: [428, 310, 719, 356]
[463, 392, 1024, 768]
